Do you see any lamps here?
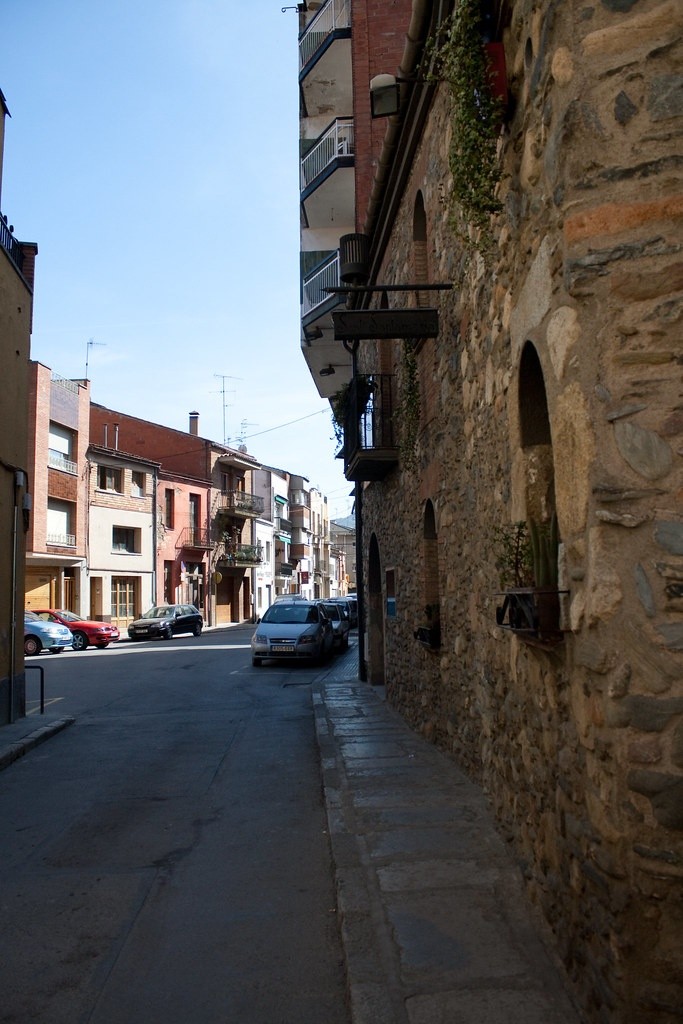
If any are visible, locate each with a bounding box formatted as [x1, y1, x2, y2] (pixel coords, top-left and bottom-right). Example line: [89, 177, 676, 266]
[369, 74, 415, 120]
[320, 365, 336, 378]
[329, 390, 342, 402]
[306, 326, 323, 344]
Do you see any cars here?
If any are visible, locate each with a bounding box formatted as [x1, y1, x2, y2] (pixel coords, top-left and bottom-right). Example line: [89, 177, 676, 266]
[274, 594, 308, 610]
[128, 605, 203, 641]
[250, 600, 334, 670]
[24, 610, 74, 656]
[30, 609, 120, 651]
[326, 593, 358, 628]
[324, 603, 349, 654]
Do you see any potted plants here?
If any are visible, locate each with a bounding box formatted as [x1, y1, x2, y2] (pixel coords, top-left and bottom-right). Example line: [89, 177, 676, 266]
[419, 602, 441, 646]
[486, 514, 564, 634]
